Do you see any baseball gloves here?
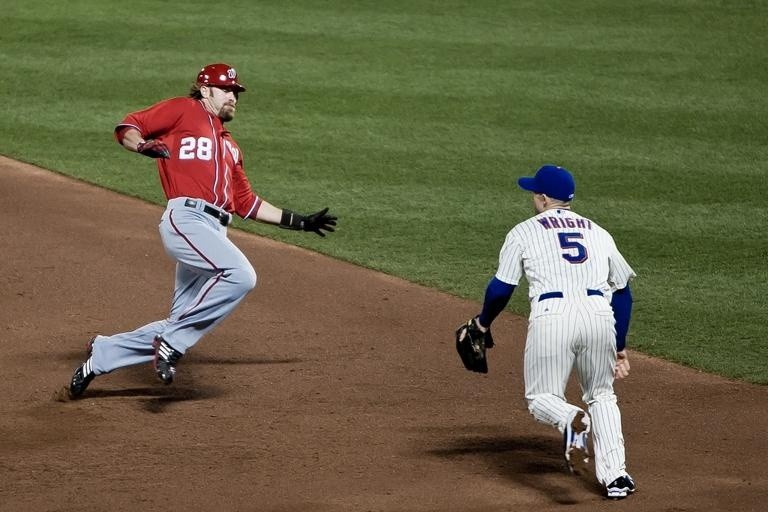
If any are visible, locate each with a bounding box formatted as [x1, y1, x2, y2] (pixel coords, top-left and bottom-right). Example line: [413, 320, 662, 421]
[455, 316, 495, 374]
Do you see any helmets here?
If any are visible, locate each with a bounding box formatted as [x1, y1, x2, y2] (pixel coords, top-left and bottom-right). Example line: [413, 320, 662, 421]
[197, 63, 245, 90]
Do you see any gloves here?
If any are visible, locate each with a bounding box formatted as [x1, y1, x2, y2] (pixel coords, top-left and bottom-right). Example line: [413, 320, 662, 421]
[279, 206, 338, 237]
[137, 139, 171, 159]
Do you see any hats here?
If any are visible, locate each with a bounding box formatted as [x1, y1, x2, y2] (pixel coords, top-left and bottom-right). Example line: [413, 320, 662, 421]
[516, 163, 574, 200]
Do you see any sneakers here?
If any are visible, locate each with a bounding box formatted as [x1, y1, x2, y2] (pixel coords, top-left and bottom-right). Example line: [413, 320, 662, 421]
[152, 333, 183, 385]
[605, 474, 636, 497]
[70, 335, 103, 395]
[563, 412, 591, 474]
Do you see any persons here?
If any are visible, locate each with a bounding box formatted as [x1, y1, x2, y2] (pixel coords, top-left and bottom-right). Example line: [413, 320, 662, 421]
[70, 63, 338, 400]
[454, 164, 637, 501]
